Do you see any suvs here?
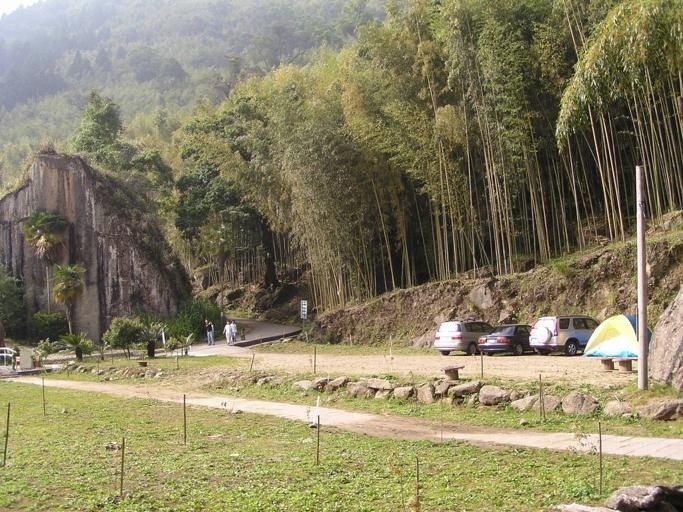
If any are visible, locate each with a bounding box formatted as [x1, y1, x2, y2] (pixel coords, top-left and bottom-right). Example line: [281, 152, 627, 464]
[527, 314, 600, 357]
[434, 322, 492, 357]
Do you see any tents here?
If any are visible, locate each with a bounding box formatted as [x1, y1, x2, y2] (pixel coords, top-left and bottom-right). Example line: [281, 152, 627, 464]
[582, 312, 652, 358]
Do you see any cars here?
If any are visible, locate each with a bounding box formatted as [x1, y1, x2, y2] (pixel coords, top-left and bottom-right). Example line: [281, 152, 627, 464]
[478, 323, 534, 355]
[0, 348, 17, 364]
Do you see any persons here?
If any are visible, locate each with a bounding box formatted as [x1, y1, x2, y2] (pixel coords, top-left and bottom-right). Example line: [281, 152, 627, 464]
[205, 319, 215, 346]
[223, 320, 232, 344]
[230, 320, 237, 343]
